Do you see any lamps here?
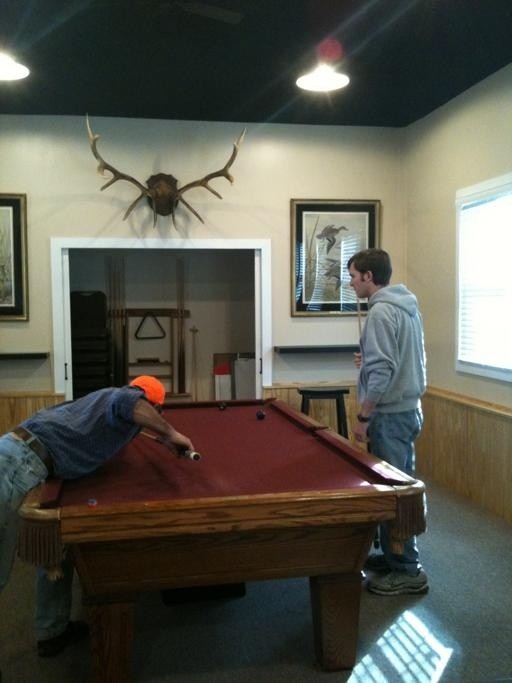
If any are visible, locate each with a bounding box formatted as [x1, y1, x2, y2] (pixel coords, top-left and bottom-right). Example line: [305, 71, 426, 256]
[0, 50, 30, 81]
[296, 63, 351, 92]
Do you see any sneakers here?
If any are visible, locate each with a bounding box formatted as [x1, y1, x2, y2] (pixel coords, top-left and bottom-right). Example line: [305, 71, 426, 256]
[365, 553, 428, 595]
[37, 620, 90, 657]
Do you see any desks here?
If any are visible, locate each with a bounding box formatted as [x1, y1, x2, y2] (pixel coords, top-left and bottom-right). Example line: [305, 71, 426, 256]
[16, 397, 428, 683]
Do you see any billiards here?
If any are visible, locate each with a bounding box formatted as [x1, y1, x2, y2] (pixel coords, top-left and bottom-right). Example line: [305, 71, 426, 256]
[85, 498, 98, 507]
[217, 400, 226, 410]
[45, 564, 64, 585]
[391, 541, 404, 555]
[256, 408, 266, 420]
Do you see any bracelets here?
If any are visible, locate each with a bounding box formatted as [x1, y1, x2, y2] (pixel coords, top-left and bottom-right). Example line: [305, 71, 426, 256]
[356, 410, 372, 424]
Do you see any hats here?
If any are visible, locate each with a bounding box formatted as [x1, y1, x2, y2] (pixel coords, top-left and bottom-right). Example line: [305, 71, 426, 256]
[129, 375, 166, 405]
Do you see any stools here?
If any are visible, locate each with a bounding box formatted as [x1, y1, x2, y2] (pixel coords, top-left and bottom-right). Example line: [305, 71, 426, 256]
[297, 386, 350, 439]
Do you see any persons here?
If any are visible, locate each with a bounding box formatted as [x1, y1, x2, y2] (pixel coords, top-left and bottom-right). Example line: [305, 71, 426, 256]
[1, 374, 194, 659]
[346, 247, 428, 596]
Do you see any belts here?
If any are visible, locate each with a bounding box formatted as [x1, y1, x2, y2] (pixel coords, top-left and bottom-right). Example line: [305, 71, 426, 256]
[11, 426, 53, 472]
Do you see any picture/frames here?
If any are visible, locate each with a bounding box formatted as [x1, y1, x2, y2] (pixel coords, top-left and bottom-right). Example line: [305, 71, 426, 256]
[0, 193, 29, 322]
[290, 198, 381, 317]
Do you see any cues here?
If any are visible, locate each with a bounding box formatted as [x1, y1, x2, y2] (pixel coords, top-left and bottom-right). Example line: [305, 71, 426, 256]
[139, 431, 201, 462]
[104, 254, 129, 388]
[175, 259, 186, 394]
[356, 299, 362, 335]
[189, 325, 198, 401]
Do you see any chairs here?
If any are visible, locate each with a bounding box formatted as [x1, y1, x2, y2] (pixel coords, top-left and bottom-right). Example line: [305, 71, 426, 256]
[70, 290, 113, 401]
